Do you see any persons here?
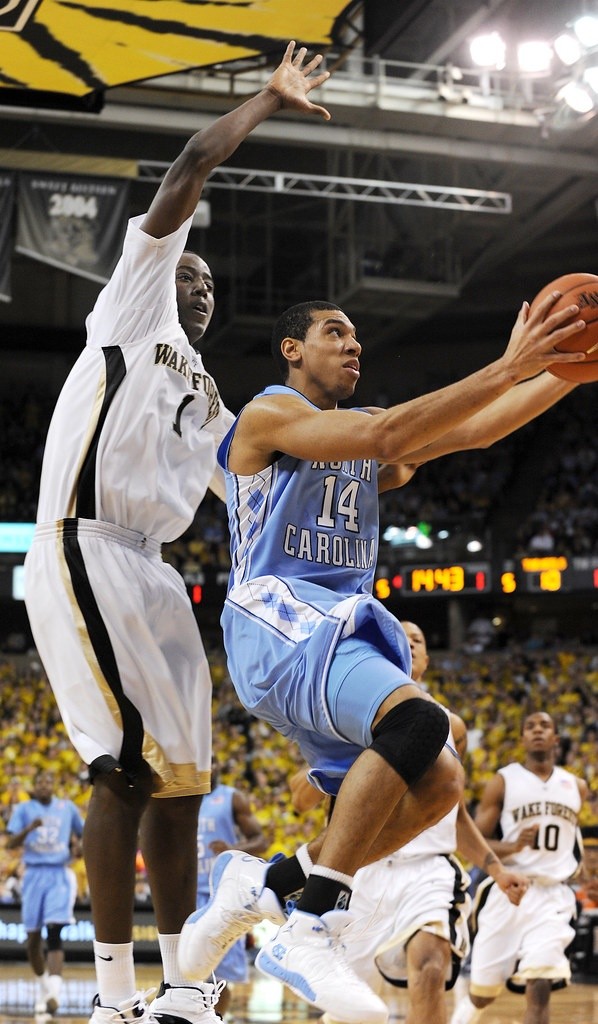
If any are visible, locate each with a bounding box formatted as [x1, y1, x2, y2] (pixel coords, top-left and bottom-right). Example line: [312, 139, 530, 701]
[1, 380, 598, 1024]
[177, 290, 585, 1024]
[23, 40, 425, 1024]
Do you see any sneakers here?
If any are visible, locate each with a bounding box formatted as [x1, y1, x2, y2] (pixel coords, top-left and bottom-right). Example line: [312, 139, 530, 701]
[173, 850, 287, 986]
[85, 988, 162, 1024]
[252, 908, 390, 1024]
[147, 978, 228, 1024]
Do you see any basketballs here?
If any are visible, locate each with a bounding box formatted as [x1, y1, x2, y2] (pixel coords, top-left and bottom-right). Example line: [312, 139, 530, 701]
[527, 273, 598, 384]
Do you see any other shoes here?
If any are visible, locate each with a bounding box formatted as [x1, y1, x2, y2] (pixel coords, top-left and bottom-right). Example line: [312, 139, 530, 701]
[32, 984, 60, 1015]
